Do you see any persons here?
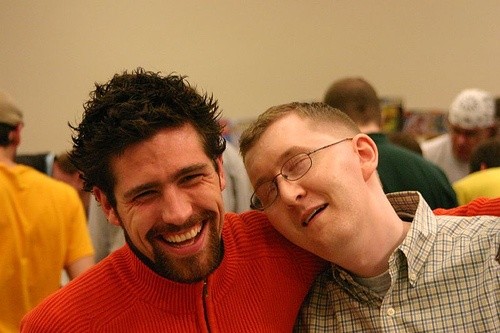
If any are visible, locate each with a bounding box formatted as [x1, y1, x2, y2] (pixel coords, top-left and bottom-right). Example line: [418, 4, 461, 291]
[88, 131, 255, 264]
[239, 100, 500, 332]
[21, 148, 88, 193]
[0, 89, 96, 333]
[323, 76, 500, 210]
[19, 64, 500, 332]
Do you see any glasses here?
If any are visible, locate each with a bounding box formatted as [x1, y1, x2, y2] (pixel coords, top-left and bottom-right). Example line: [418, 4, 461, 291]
[250, 137, 353, 212]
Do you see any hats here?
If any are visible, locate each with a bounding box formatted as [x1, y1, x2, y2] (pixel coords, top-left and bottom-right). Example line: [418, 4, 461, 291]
[0, 87, 24, 126]
[448, 88, 496, 129]
